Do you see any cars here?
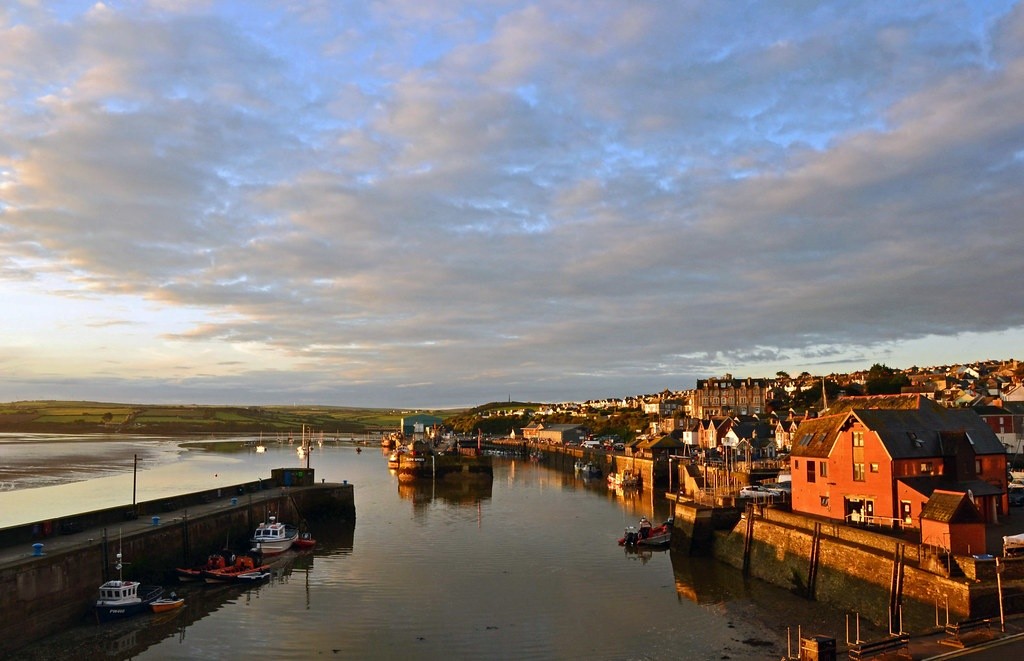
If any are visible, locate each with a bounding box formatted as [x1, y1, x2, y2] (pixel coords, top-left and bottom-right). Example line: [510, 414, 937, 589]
[763, 483, 790, 494]
[740, 485, 780, 500]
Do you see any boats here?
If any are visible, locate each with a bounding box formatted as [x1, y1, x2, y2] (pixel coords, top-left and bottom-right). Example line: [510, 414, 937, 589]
[528, 449, 639, 487]
[296, 533, 317, 547]
[201, 553, 255, 585]
[176, 554, 228, 582]
[296, 444, 314, 456]
[94, 528, 166, 621]
[256, 445, 267, 452]
[150, 597, 188, 612]
[622, 517, 672, 548]
[249, 511, 298, 553]
[238, 569, 272, 586]
